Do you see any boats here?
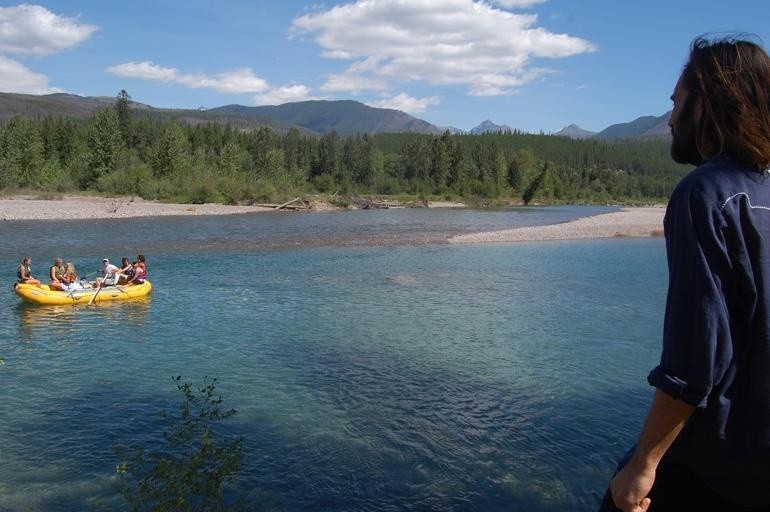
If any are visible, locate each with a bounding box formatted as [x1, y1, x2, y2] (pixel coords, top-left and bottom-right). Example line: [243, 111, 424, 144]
[7, 278, 154, 303]
[20, 296, 152, 334]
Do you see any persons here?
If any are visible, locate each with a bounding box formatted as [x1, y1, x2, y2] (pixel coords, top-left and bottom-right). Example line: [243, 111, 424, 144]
[600, 35, 770, 512]
[17, 257, 41, 288]
[50, 255, 146, 291]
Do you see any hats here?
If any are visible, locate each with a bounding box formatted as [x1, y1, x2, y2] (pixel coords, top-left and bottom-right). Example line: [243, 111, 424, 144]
[103, 259, 109, 262]
